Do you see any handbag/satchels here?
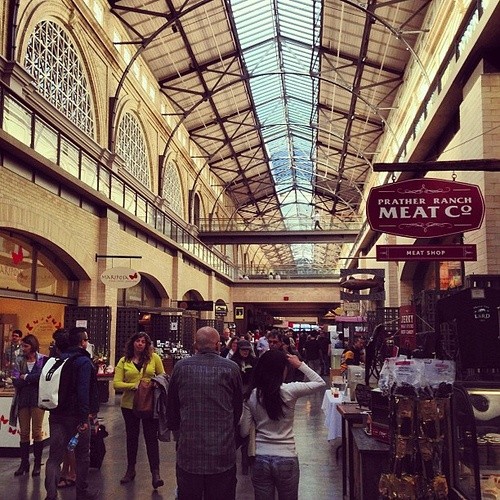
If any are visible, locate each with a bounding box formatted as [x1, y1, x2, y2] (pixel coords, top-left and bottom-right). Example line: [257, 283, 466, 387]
[133, 380, 153, 420]
[91, 422, 109, 469]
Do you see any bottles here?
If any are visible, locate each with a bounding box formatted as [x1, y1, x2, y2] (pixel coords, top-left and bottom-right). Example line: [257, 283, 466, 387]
[68, 432, 81, 450]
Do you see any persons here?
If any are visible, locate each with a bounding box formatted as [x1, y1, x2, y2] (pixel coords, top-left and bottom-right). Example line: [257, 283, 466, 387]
[167, 326, 245, 500]
[240, 349, 326, 500]
[340, 334, 363, 376]
[220, 328, 333, 383]
[335, 333, 346, 349]
[4, 330, 48, 476]
[43, 327, 99, 500]
[112, 332, 166, 488]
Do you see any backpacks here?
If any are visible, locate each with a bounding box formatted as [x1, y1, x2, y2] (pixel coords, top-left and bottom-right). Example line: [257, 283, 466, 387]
[37, 355, 71, 412]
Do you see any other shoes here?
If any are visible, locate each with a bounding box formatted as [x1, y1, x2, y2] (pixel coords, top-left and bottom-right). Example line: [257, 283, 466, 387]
[56, 478, 76, 488]
[76, 493, 97, 500]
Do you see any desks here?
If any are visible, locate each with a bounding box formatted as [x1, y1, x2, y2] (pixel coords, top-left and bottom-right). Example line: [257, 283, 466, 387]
[322, 389, 391, 500]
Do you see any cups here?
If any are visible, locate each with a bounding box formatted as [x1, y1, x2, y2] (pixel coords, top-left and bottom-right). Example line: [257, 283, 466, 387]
[331, 387, 335, 394]
[334, 388, 339, 398]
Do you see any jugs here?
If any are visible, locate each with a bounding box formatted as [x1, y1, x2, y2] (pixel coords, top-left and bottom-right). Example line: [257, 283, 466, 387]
[98, 364, 106, 374]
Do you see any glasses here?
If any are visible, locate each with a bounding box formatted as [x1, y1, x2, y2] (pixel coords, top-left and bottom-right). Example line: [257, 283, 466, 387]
[268, 340, 281, 344]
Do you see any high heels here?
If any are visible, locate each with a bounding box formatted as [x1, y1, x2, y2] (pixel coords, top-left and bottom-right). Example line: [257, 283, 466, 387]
[120, 464, 136, 483]
[152, 471, 163, 489]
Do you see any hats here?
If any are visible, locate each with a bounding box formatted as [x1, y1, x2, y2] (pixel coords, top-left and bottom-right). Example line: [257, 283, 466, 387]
[237, 340, 252, 349]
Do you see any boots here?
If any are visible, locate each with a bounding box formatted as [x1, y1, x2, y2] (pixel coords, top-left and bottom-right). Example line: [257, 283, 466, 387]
[14, 441, 30, 476]
[32, 440, 45, 476]
